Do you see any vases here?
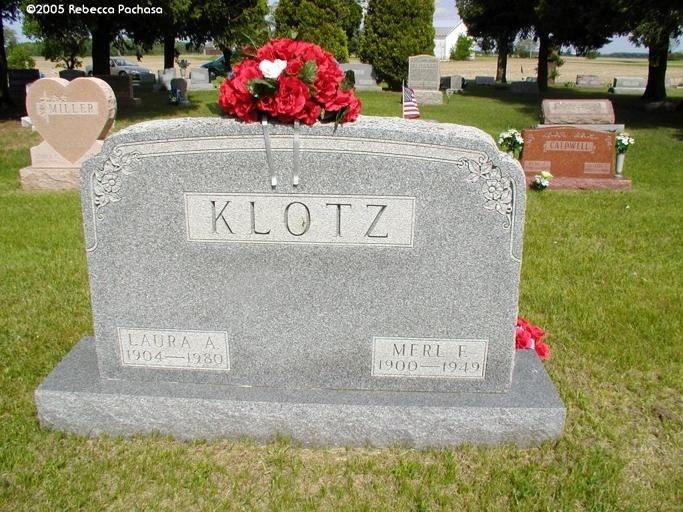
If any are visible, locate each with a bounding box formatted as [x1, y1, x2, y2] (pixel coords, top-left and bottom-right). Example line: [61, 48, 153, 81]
[616, 152, 625, 174]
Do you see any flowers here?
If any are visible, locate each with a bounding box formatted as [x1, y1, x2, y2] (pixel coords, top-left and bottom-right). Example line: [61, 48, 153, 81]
[513, 316, 550, 359]
[496, 124, 523, 159]
[217, 26, 362, 137]
[614, 133, 634, 155]
[534, 170, 554, 192]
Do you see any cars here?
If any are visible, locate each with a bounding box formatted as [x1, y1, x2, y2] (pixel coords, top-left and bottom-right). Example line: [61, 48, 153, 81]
[201, 53, 254, 81]
[85, 58, 151, 80]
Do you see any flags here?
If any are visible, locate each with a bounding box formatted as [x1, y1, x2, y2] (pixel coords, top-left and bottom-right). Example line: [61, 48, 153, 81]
[401, 78, 421, 119]
